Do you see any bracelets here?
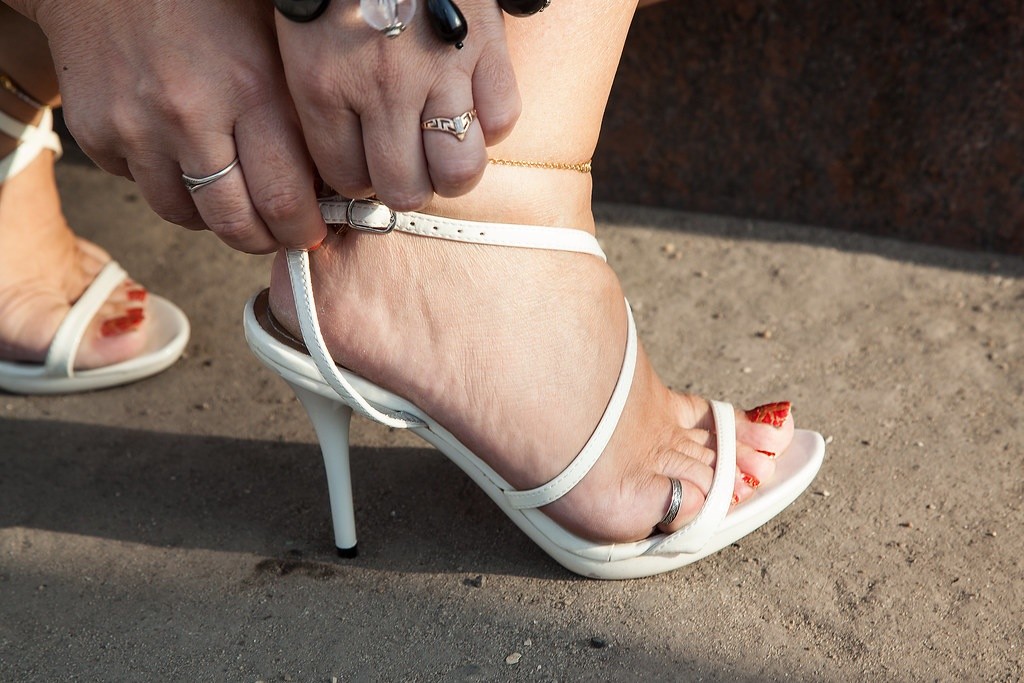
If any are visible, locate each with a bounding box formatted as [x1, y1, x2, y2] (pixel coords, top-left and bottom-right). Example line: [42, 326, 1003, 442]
[275, 0, 551, 49]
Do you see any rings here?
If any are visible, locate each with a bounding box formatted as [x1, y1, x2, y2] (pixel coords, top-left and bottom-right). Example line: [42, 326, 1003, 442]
[421, 108, 477, 141]
[183, 158, 238, 192]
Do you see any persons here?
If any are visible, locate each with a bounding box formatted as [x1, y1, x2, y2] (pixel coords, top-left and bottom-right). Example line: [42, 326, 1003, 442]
[0, 0, 826, 578]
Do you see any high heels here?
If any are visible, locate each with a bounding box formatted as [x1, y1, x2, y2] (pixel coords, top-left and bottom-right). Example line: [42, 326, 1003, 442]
[240, 196, 826, 582]
[0, 103, 193, 396]
